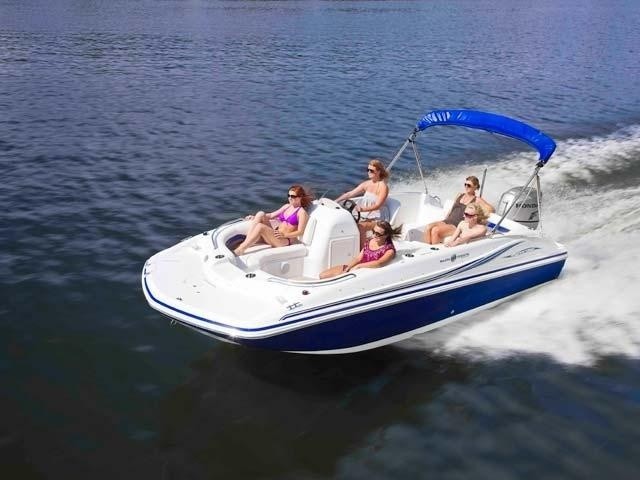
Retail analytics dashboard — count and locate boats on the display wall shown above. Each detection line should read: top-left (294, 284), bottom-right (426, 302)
top-left (142, 109), bottom-right (568, 356)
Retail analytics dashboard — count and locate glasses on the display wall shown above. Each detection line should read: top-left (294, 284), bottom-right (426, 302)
top-left (464, 212), bottom-right (475, 218)
top-left (371, 228), bottom-right (384, 237)
top-left (464, 182), bottom-right (476, 188)
top-left (367, 168), bottom-right (379, 173)
top-left (287, 194), bottom-right (300, 198)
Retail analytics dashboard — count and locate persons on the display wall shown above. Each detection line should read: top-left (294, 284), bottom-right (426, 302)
top-left (332, 159), bottom-right (392, 250)
top-left (423, 175), bottom-right (497, 245)
top-left (318, 219), bottom-right (403, 280)
top-left (442, 202), bottom-right (488, 247)
top-left (230, 184), bottom-right (316, 257)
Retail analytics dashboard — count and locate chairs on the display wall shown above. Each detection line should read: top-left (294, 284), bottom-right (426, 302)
top-left (242, 215), bottom-right (317, 277)
top-left (366, 197), bottom-right (401, 237)
